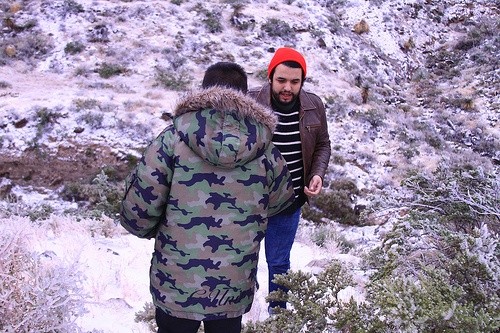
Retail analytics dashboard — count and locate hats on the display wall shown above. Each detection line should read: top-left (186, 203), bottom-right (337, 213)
top-left (267, 48), bottom-right (306, 81)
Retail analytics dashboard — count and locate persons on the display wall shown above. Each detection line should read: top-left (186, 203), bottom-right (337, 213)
top-left (248, 48), bottom-right (331, 315)
top-left (119, 62), bottom-right (296, 333)
